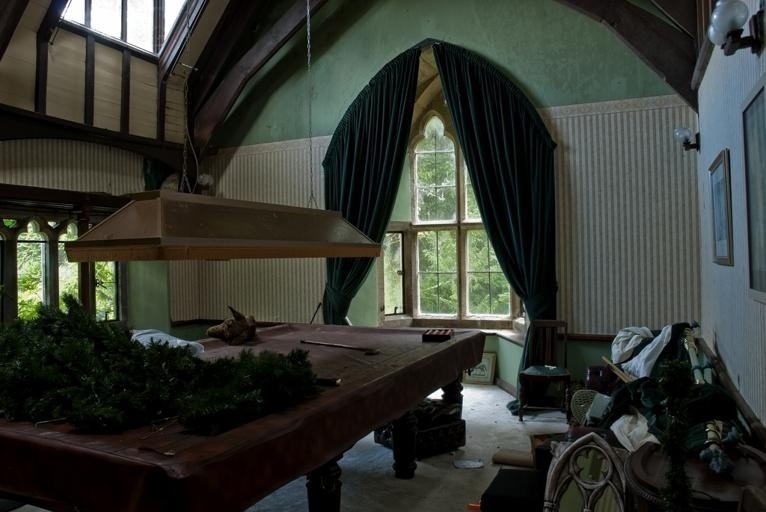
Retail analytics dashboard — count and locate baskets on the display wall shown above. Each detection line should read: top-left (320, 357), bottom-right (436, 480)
top-left (570, 389), bottom-right (599, 426)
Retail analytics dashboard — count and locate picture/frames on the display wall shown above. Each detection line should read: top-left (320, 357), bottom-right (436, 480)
top-left (463, 353), bottom-right (496, 385)
top-left (708, 147), bottom-right (734, 267)
top-left (738, 73), bottom-right (766, 304)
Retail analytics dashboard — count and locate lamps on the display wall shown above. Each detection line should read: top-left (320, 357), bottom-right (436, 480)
top-left (674, 125), bottom-right (701, 153)
top-left (705, 0), bottom-right (764, 55)
top-left (66, 1), bottom-right (380, 262)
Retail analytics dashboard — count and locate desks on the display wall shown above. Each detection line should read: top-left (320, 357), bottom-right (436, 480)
top-left (0, 320), bottom-right (487, 512)
top-left (623, 436), bottom-right (766, 512)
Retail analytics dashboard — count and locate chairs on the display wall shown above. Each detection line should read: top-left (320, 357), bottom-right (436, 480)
top-left (518, 319), bottom-right (573, 424)
